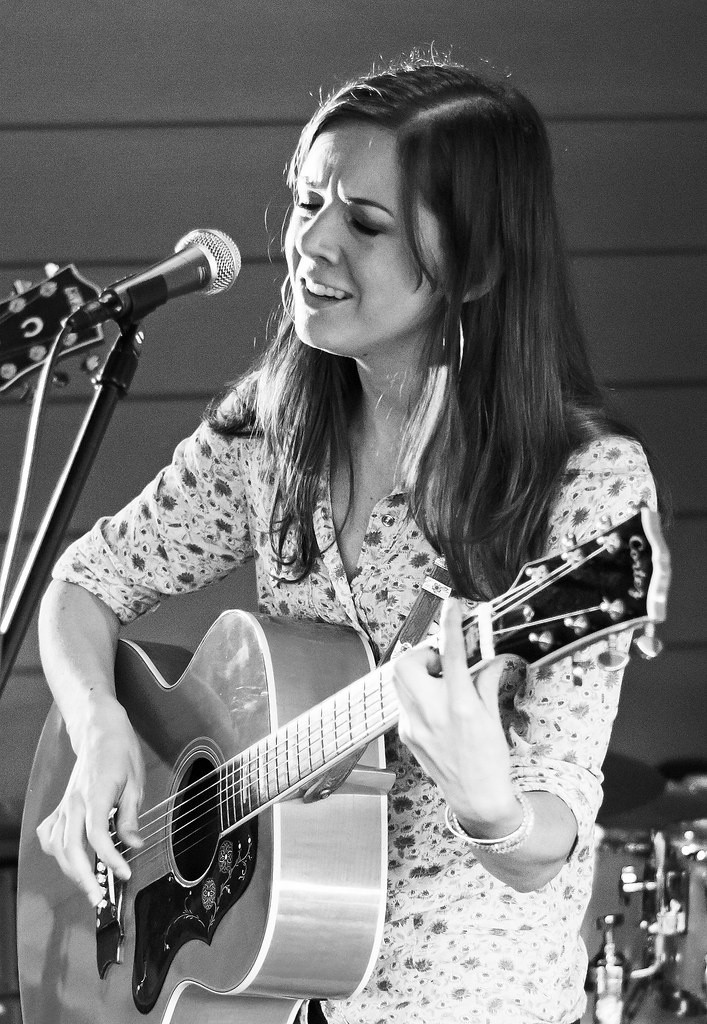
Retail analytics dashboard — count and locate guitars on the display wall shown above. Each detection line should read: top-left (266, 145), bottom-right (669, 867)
top-left (8, 499), bottom-right (681, 1024)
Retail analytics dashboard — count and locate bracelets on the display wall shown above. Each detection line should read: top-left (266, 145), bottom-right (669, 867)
top-left (446, 787), bottom-right (535, 853)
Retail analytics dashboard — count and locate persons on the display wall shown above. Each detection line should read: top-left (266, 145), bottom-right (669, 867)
top-left (37, 61), bottom-right (659, 1024)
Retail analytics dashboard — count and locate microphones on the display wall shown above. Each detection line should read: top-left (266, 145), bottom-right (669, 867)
top-left (67, 229), bottom-right (241, 333)
